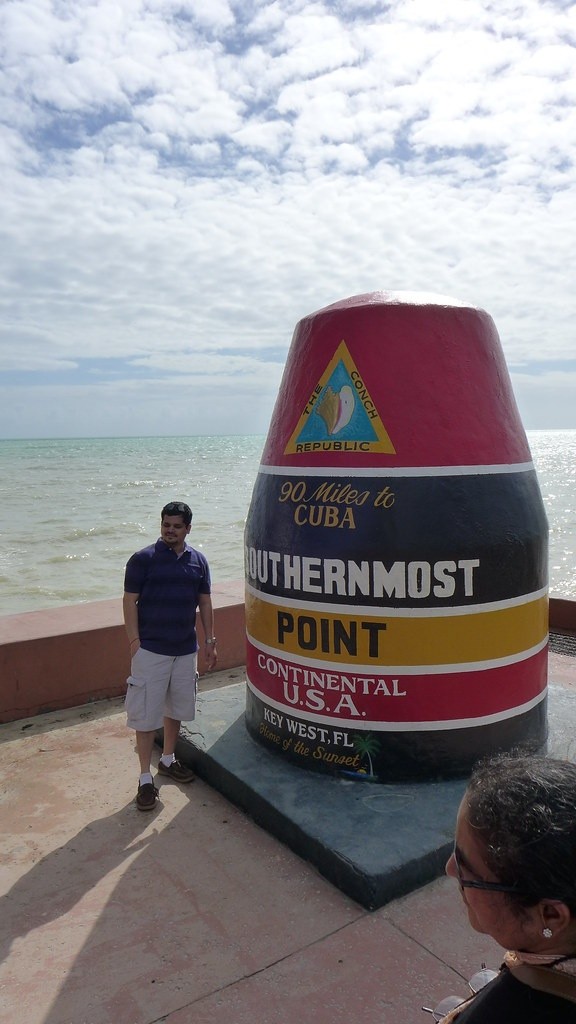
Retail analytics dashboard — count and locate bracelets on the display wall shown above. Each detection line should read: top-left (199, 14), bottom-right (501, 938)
top-left (205, 636), bottom-right (217, 644)
top-left (129, 638), bottom-right (140, 645)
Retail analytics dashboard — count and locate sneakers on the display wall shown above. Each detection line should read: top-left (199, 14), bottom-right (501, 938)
top-left (137, 779), bottom-right (157, 811)
top-left (158, 760), bottom-right (195, 783)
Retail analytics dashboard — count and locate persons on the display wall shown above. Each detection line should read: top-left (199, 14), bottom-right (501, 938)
top-left (123, 502), bottom-right (215, 810)
top-left (435, 746), bottom-right (576, 1024)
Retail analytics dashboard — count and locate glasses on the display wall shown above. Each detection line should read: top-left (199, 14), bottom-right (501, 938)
top-left (453, 839), bottom-right (567, 902)
top-left (166, 503), bottom-right (184, 512)
